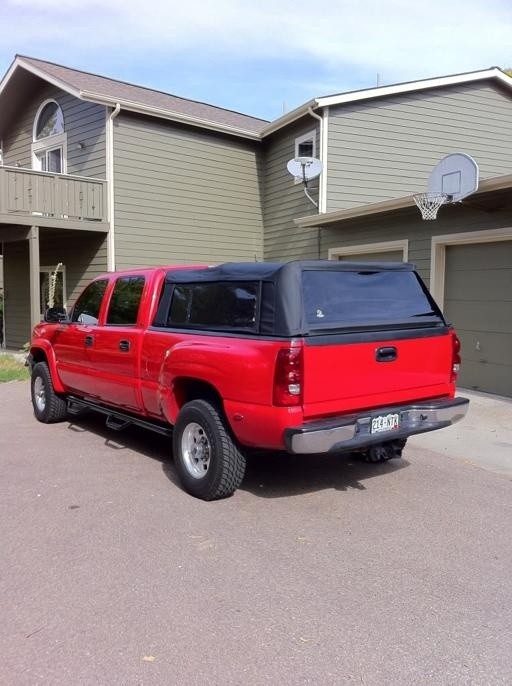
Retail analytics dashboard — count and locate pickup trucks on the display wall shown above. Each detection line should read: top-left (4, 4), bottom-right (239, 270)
top-left (25, 257), bottom-right (472, 502)
